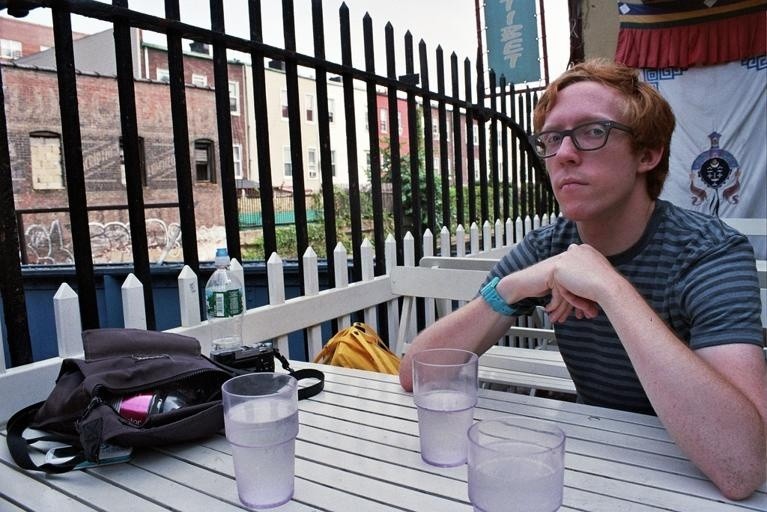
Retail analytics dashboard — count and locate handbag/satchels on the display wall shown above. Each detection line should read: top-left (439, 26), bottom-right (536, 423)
top-left (6, 327), bottom-right (228, 474)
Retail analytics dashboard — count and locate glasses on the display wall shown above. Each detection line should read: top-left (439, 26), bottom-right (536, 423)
top-left (528, 120), bottom-right (634, 159)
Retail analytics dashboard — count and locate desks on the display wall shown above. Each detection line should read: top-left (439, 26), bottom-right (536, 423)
top-left (0, 349), bottom-right (767, 512)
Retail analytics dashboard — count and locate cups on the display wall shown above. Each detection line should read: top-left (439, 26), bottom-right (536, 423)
top-left (224, 372), bottom-right (299, 508)
top-left (410, 349), bottom-right (480, 464)
top-left (468, 420), bottom-right (565, 512)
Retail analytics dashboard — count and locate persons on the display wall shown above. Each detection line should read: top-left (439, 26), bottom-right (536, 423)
top-left (400, 58), bottom-right (767, 500)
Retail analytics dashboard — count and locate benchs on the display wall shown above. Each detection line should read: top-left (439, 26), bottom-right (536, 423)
top-left (386, 247), bottom-right (580, 399)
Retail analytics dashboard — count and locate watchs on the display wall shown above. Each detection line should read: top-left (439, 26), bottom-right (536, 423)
top-left (480, 276), bottom-right (516, 316)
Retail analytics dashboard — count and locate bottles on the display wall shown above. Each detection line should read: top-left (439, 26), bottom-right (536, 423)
top-left (204, 248), bottom-right (244, 350)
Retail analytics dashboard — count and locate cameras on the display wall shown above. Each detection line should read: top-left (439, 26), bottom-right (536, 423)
top-left (210, 344), bottom-right (274, 376)
